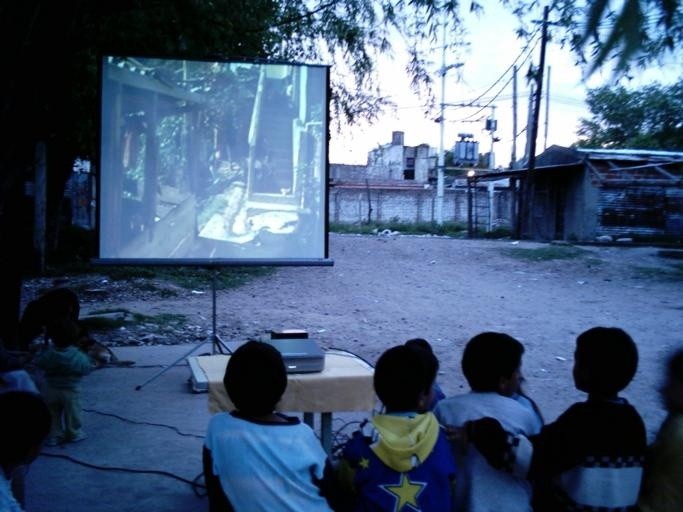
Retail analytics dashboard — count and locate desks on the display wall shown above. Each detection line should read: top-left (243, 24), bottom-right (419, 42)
top-left (187, 351), bottom-right (374, 462)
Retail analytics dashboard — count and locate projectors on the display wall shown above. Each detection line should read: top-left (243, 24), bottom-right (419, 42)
top-left (262, 338), bottom-right (326, 373)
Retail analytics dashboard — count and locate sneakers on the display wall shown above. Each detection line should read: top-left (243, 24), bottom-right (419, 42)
top-left (67, 428), bottom-right (88, 444)
top-left (44, 435), bottom-right (64, 447)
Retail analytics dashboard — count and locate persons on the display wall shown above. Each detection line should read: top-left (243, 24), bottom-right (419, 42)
top-left (433, 331), bottom-right (546, 510)
top-left (332, 337), bottom-right (472, 511)
top-left (0, 286), bottom-right (88, 510)
top-left (641, 346), bottom-right (681, 511)
top-left (201, 339), bottom-right (334, 510)
top-left (460, 325), bottom-right (649, 512)
